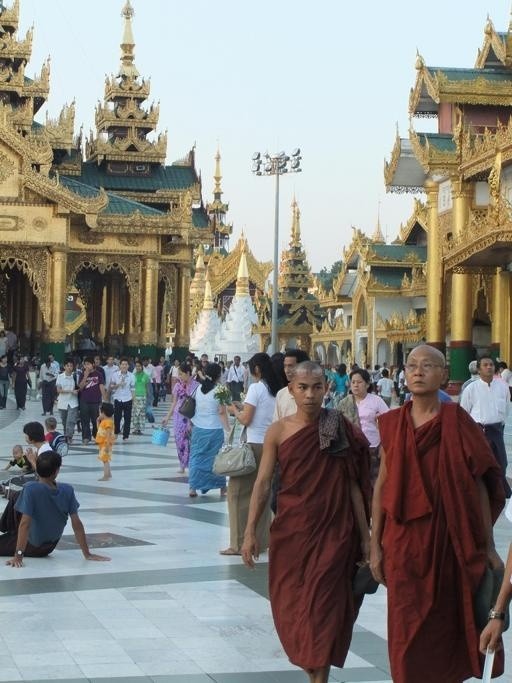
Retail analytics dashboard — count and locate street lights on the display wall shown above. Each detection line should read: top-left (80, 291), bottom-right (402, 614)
top-left (251, 144), bottom-right (306, 358)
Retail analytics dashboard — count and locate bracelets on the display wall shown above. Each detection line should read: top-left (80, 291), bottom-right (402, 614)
top-left (486, 609), bottom-right (506, 620)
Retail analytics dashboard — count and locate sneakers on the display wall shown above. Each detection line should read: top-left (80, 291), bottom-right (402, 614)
top-left (67, 427), bottom-right (144, 446)
top-left (1, 405), bottom-right (54, 417)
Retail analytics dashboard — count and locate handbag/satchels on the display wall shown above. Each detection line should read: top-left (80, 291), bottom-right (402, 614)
top-left (145, 407), bottom-right (155, 423)
top-left (212, 444), bottom-right (256, 478)
top-left (472, 564), bottom-right (508, 634)
top-left (150, 426), bottom-right (170, 448)
top-left (179, 397), bottom-right (197, 418)
top-left (158, 390), bottom-right (167, 399)
top-left (353, 562), bottom-right (381, 597)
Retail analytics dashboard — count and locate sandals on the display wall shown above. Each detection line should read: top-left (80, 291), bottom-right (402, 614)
top-left (219, 544), bottom-right (247, 556)
top-left (188, 486), bottom-right (228, 497)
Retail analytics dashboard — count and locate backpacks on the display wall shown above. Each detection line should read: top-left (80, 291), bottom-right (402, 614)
top-left (48, 433), bottom-right (69, 457)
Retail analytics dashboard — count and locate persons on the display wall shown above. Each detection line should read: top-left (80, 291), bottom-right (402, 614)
top-left (367, 346), bottom-right (512, 683)
top-left (241, 362), bottom-right (378, 683)
top-left (480, 546), bottom-right (512, 654)
top-left (2, 327), bottom-right (512, 567)
